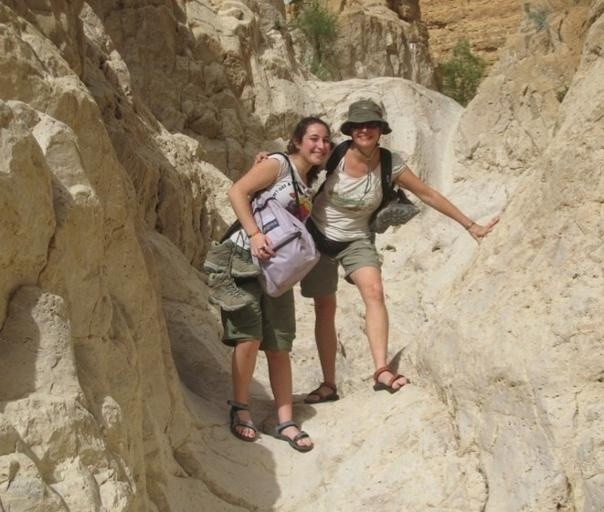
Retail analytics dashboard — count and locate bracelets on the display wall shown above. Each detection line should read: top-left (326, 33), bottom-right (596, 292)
top-left (465, 221), bottom-right (475, 229)
top-left (246, 228), bottom-right (261, 238)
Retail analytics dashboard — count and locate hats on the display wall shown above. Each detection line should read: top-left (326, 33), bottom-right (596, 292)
top-left (340, 100), bottom-right (392, 136)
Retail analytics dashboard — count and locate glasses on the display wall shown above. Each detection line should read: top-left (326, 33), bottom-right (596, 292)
top-left (349, 122), bottom-right (381, 128)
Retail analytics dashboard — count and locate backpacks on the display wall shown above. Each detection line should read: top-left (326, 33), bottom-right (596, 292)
top-left (241, 152), bottom-right (320, 298)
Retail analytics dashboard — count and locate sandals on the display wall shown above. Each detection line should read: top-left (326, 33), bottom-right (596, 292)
top-left (304, 383), bottom-right (339, 403)
top-left (373, 367), bottom-right (410, 392)
top-left (226, 399), bottom-right (257, 441)
top-left (274, 421), bottom-right (313, 451)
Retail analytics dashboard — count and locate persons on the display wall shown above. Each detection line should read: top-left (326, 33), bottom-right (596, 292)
top-left (253, 99), bottom-right (501, 405)
top-left (205, 114), bottom-right (329, 452)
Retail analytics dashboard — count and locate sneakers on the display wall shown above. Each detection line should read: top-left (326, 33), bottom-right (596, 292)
top-left (372, 189), bottom-right (420, 233)
top-left (207, 272), bottom-right (253, 312)
top-left (203, 239), bottom-right (261, 277)
